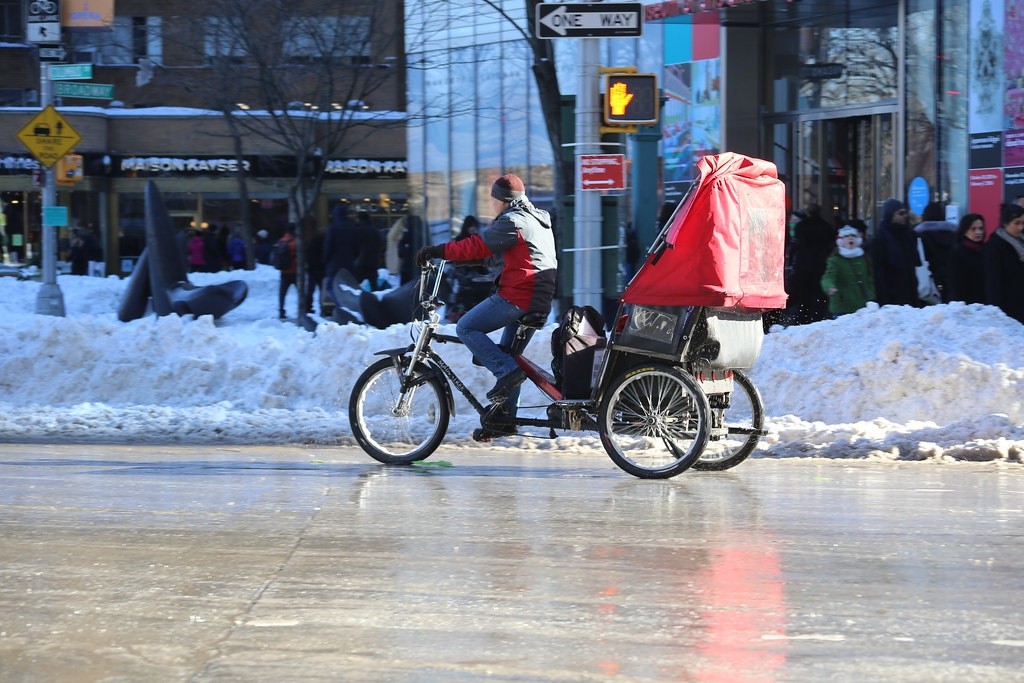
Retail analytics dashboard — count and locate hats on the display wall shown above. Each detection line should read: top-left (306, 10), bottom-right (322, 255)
top-left (491, 174), bottom-right (525, 203)
top-left (257, 229), bottom-right (269, 238)
top-left (879, 198), bottom-right (906, 223)
top-left (923, 200), bottom-right (950, 216)
top-left (792, 208), bottom-right (811, 219)
top-left (835, 223), bottom-right (859, 237)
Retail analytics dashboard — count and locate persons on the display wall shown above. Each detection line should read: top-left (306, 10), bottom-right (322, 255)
top-left (278, 202), bottom-right (387, 319)
top-left (455, 212), bottom-right (489, 276)
top-left (178, 220), bottom-right (250, 271)
top-left (67, 220), bottom-right (101, 276)
top-left (985, 202), bottom-right (1024, 324)
top-left (118, 227), bottom-right (132, 256)
top-left (943, 212), bottom-right (996, 307)
top-left (788, 201), bottom-right (874, 322)
top-left (867, 196), bottom-right (931, 309)
top-left (399, 215), bottom-right (431, 284)
top-left (624, 221), bottom-right (641, 280)
top-left (913, 199), bottom-right (961, 292)
top-left (248, 229), bottom-right (279, 269)
top-left (415, 173), bottom-right (559, 436)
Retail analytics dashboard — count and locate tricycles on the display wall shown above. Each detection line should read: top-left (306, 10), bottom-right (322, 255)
top-left (349, 151), bottom-right (789, 480)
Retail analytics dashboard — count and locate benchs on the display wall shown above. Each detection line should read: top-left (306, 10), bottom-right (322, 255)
top-left (608, 152), bottom-right (790, 371)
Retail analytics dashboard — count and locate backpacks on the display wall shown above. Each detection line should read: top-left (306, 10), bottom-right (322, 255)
top-left (270, 237), bottom-right (297, 269)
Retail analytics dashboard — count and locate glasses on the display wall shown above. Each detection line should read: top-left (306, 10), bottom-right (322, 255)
top-left (894, 211), bottom-right (909, 216)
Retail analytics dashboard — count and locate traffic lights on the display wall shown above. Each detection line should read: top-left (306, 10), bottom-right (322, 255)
top-left (605, 73), bottom-right (657, 124)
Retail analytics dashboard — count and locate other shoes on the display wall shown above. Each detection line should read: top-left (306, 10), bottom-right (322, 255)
top-left (279, 309), bottom-right (287, 318)
top-left (308, 310), bottom-right (315, 313)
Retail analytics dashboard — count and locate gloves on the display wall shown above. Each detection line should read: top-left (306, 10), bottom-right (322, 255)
top-left (416, 243), bottom-right (445, 268)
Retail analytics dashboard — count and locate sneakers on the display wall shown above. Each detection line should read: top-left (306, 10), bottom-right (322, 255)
top-left (473, 425), bottom-right (518, 438)
top-left (486, 367), bottom-right (527, 399)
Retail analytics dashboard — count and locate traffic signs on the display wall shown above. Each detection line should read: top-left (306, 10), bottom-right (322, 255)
top-left (536, 2), bottom-right (644, 40)
